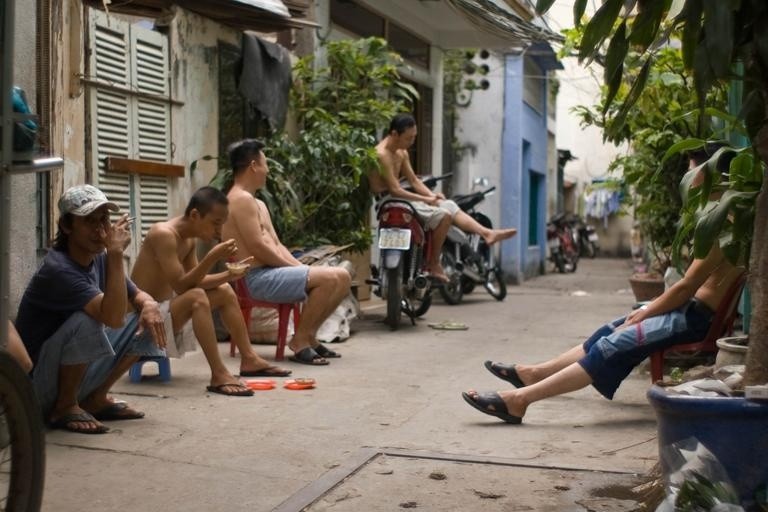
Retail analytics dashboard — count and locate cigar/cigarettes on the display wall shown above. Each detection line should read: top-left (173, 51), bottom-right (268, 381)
top-left (126, 216), bottom-right (137, 222)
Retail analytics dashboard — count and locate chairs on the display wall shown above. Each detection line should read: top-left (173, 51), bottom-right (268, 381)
top-left (226, 254), bottom-right (301, 360)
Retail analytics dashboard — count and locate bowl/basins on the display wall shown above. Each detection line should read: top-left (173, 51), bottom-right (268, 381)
top-left (227, 263), bottom-right (248, 275)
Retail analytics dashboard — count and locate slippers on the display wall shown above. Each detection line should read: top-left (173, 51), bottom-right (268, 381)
top-left (428, 319), bottom-right (469, 330)
top-left (289, 343), bottom-right (341, 365)
top-left (239, 364), bottom-right (288, 376)
top-left (206, 382), bottom-right (254, 396)
top-left (91, 398), bottom-right (145, 420)
top-left (484, 359), bottom-right (525, 388)
top-left (461, 390), bottom-right (522, 424)
top-left (48, 411), bottom-right (106, 434)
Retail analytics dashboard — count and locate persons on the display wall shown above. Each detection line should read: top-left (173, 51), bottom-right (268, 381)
top-left (370, 113), bottom-right (517, 284)
top-left (5, 317), bottom-right (34, 373)
top-left (15, 184), bottom-right (169, 434)
top-left (221, 140), bottom-right (352, 366)
top-left (127, 187), bottom-right (292, 397)
top-left (463, 141), bottom-right (746, 423)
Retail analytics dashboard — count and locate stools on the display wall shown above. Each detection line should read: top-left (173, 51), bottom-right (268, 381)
top-left (130, 356), bottom-right (170, 383)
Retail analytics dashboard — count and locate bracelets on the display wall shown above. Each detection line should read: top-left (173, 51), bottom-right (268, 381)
top-left (640, 305), bottom-right (647, 311)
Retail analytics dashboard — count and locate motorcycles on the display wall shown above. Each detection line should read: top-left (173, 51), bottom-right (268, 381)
top-left (547, 212), bottom-right (599, 272)
top-left (437, 186), bottom-right (506, 303)
top-left (365, 173), bottom-right (453, 325)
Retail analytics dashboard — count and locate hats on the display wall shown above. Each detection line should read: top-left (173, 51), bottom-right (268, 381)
top-left (58, 185), bottom-right (119, 218)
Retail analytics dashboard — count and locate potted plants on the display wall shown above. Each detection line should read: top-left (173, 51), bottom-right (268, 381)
top-left (531, 0), bottom-right (768, 512)
